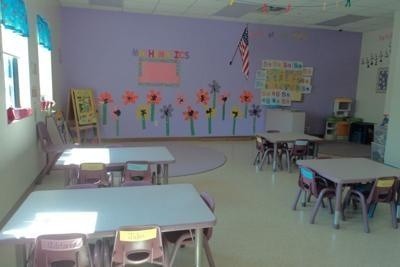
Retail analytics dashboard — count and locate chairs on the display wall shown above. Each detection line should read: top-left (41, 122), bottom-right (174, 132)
top-left (291, 163), bottom-right (336, 224)
top-left (121, 160), bottom-right (156, 186)
top-left (342, 176), bottom-right (398, 233)
top-left (36, 121), bottom-right (80, 184)
top-left (102, 225), bottom-right (168, 267)
top-left (164, 192), bottom-right (215, 267)
top-left (79, 163), bottom-right (112, 188)
top-left (30, 233), bottom-right (101, 267)
top-left (253, 134), bottom-right (283, 170)
top-left (286, 138), bottom-right (309, 171)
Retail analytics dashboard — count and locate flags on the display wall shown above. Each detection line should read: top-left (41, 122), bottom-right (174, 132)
top-left (238, 25), bottom-right (249, 79)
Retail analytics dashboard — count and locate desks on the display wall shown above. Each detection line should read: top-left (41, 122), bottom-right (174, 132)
top-left (295, 157), bottom-right (400, 228)
top-left (255, 133), bottom-right (324, 172)
top-left (53, 145), bottom-right (175, 185)
top-left (0, 183), bottom-right (216, 266)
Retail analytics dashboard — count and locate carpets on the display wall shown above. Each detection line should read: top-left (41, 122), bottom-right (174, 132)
top-left (47, 140), bottom-right (227, 177)
